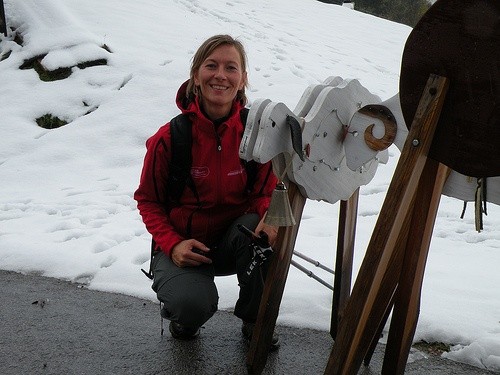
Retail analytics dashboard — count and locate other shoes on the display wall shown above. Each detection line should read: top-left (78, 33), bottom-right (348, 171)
top-left (169, 321), bottom-right (191, 339)
top-left (242, 320), bottom-right (279, 348)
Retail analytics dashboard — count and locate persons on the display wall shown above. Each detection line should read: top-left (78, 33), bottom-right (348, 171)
top-left (133, 34), bottom-right (294, 345)
